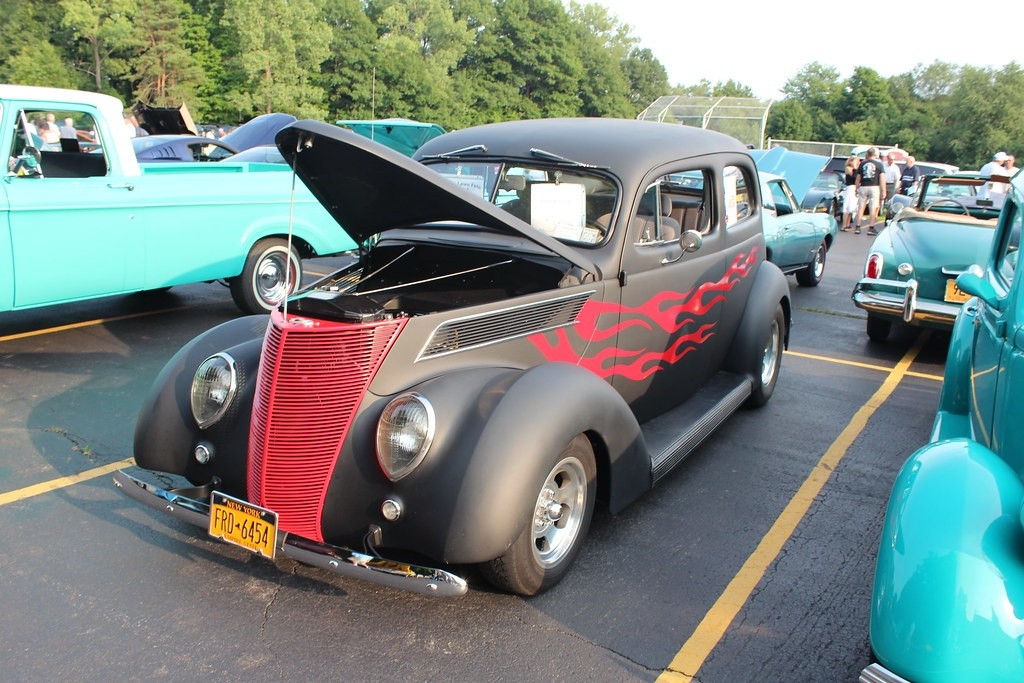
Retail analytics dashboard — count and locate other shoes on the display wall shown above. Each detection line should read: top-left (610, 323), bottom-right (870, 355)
top-left (841, 226), bottom-right (853, 231)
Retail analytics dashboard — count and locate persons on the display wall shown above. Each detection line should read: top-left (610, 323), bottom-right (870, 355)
top-left (841, 147), bottom-right (919, 235)
top-left (25, 114), bottom-right (148, 153)
top-left (979, 152), bottom-right (1020, 191)
top-left (206, 128), bottom-right (227, 146)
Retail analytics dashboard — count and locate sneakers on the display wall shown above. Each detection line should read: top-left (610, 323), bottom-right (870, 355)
top-left (867, 228), bottom-right (878, 236)
top-left (855, 228), bottom-right (861, 234)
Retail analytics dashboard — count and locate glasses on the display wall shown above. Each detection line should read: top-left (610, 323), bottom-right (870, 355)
top-left (888, 158), bottom-right (895, 160)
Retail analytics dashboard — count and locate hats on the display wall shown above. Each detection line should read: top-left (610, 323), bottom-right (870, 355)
top-left (993, 152), bottom-right (1009, 161)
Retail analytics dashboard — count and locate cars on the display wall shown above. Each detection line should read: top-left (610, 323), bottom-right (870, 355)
top-left (851, 172), bottom-right (1011, 341)
top-left (655, 146), bottom-right (840, 287)
top-left (800, 145), bottom-right (979, 227)
top-left (0, 82), bottom-right (363, 314)
top-left (858, 167), bottom-right (1024, 683)
top-left (112, 116), bottom-right (793, 599)
